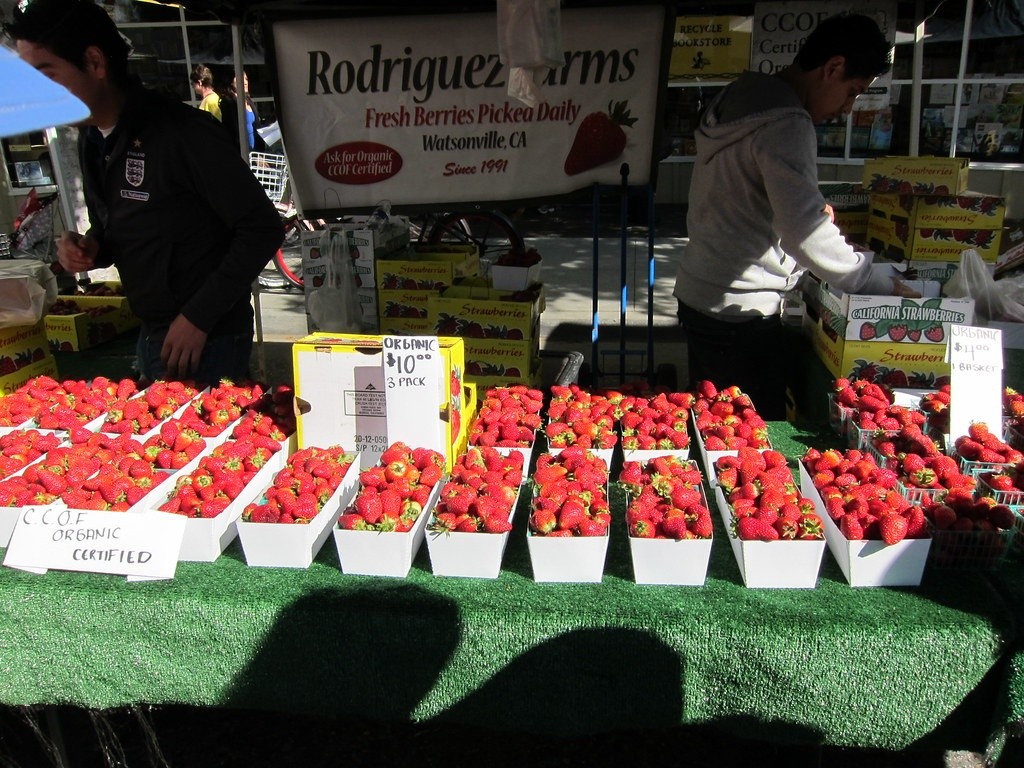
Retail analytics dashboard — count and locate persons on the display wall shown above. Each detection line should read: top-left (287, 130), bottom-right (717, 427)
top-left (189, 63), bottom-right (222, 122)
top-left (672, 14), bottom-right (923, 420)
top-left (219, 69), bottom-right (271, 169)
top-left (10, 1), bottom-right (286, 386)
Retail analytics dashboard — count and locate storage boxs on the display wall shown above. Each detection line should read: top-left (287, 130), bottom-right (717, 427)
top-left (3, 160), bottom-right (1022, 592)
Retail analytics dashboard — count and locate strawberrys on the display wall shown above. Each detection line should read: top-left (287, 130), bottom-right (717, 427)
top-left (0, 375), bottom-right (1023, 577)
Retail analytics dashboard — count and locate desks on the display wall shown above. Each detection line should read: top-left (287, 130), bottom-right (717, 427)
top-left (2, 410), bottom-right (1024, 768)
